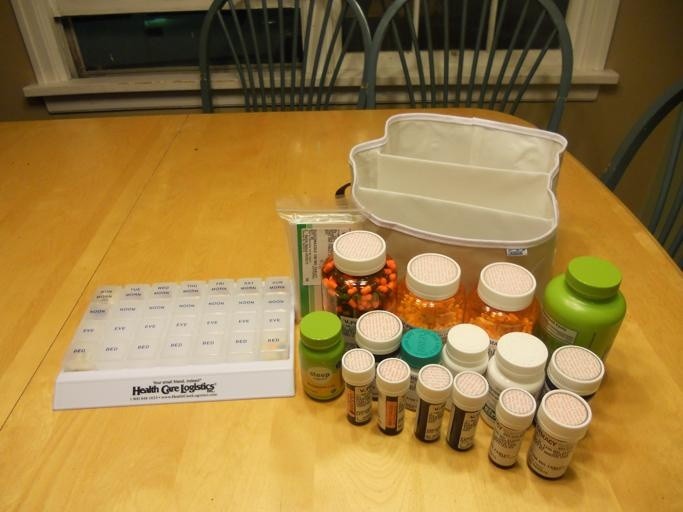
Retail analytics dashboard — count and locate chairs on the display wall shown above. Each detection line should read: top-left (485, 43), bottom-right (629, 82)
top-left (366, 0), bottom-right (575, 134)
top-left (198, 0), bottom-right (373, 107)
top-left (600, 81), bottom-right (683, 271)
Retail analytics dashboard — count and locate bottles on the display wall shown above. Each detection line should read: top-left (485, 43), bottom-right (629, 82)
top-left (297, 229), bottom-right (628, 480)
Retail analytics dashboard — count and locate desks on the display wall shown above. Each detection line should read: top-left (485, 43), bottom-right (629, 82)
top-left (0, 106), bottom-right (682, 511)
